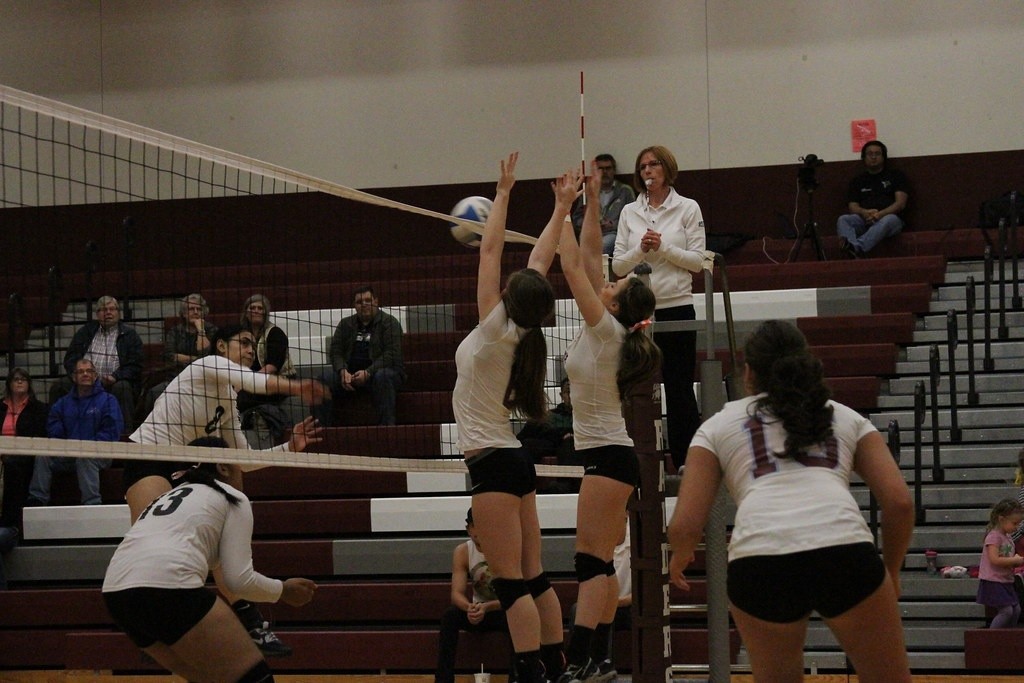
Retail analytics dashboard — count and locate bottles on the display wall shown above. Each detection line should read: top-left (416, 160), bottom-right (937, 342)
top-left (926, 552), bottom-right (937, 573)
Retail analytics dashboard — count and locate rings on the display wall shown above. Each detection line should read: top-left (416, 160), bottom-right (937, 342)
top-left (648, 238), bottom-right (653, 244)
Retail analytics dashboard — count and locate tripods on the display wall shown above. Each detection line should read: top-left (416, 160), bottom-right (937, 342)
top-left (790, 181), bottom-right (827, 264)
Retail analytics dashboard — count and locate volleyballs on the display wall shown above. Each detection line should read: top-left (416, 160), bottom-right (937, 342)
top-left (449, 196), bottom-right (494, 250)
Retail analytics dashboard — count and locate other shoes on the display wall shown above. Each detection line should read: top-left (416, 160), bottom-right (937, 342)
top-left (671, 447), bottom-right (686, 474)
top-left (567, 657), bottom-right (600, 683)
top-left (840, 247), bottom-right (862, 259)
top-left (595, 659), bottom-right (617, 683)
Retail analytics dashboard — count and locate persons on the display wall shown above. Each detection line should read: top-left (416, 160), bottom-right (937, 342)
top-left (309, 286), bottom-right (408, 429)
top-left (837, 141), bottom-right (912, 261)
top-left (103, 434), bottom-right (317, 683)
top-left (666, 320), bottom-right (916, 683)
top-left (451, 151), bottom-right (579, 683)
top-left (544, 378), bottom-right (585, 464)
top-left (974, 447), bottom-right (1024, 631)
top-left (571, 153), bottom-right (634, 257)
top-left (613, 143), bottom-right (705, 475)
top-left (435, 506), bottom-right (521, 683)
top-left (0, 293), bottom-right (299, 524)
top-left (549, 159), bottom-right (661, 683)
top-left (122, 322), bottom-right (322, 657)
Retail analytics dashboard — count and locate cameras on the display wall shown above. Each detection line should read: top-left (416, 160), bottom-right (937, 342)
top-left (795, 154), bottom-right (825, 185)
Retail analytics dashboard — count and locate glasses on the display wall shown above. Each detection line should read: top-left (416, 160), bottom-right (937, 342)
top-left (640, 160), bottom-right (663, 170)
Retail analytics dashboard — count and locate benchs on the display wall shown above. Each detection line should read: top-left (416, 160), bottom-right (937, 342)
top-left (0, 225), bottom-right (1024, 672)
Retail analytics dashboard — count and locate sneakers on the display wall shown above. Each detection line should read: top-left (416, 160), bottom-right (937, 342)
top-left (247, 620), bottom-right (291, 656)
